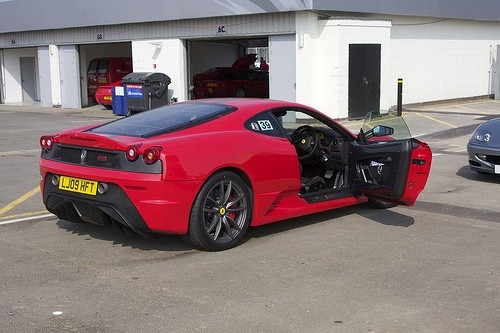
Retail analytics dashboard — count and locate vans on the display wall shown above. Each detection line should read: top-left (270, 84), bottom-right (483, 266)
top-left (86, 56), bottom-right (133, 104)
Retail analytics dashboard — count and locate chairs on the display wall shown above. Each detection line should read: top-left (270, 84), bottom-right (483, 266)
top-left (299, 155), bottom-right (324, 194)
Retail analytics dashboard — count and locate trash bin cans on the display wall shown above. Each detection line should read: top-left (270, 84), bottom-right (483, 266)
top-left (111, 71), bottom-right (171, 116)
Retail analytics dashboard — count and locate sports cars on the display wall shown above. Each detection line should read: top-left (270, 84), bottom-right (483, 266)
top-left (37, 96), bottom-right (433, 253)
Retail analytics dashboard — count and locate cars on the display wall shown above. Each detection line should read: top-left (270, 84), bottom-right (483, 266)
top-left (193, 66), bottom-right (232, 85)
top-left (192, 53), bottom-right (269, 98)
top-left (95, 79), bottom-right (123, 110)
